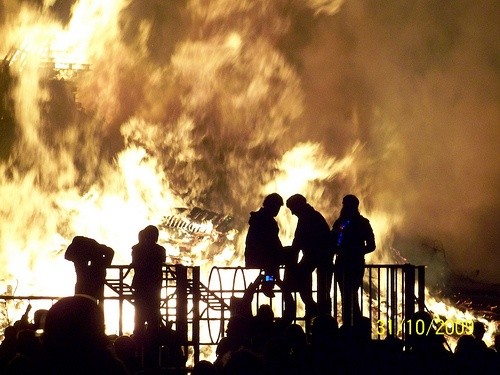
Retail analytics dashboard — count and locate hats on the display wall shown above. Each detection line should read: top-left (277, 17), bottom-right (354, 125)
top-left (342, 194), bottom-right (360, 205)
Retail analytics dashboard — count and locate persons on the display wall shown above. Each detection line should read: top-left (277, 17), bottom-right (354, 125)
top-left (65, 236), bottom-right (115, 301)
top-left (192, 273), bottom-right (500, 375)
top-left (0, 293), bottom-right (188, 375)
top-left (331, 194), bottom-right (376, 326)
top-left (131, 225), bottom-right (166, 335)
top-left (287, 194), bottom-right (332, 321)
top-left (245, 194), bottom-right (293, 302)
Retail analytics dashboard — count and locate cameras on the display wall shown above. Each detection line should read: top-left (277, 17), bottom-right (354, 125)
top-left (262, 274), bottom-right (275, 284)
